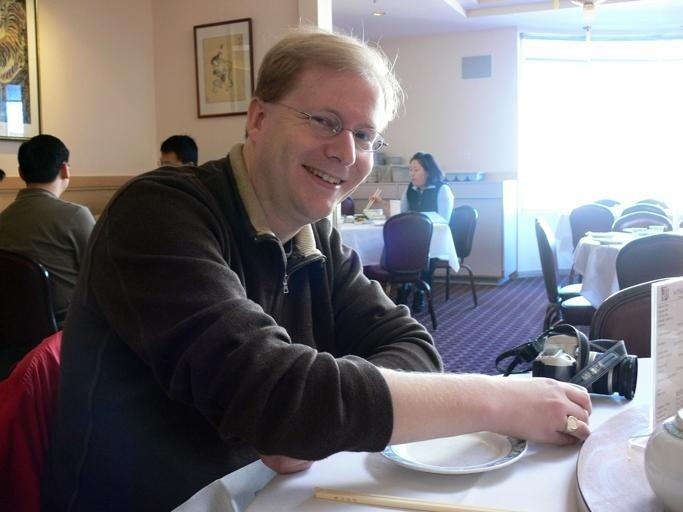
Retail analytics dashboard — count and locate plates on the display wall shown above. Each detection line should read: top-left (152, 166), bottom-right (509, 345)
top-left (378, 426), bottom-right (530, 476)
top-left (587, 229), bottom-right (656, 247)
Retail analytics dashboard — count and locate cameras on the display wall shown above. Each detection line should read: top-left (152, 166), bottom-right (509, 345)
top-left (532, 334), bottom-right (638, 400)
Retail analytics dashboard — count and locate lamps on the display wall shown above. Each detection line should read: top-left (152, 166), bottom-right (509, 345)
top-left (569, 0), bottom-right (607, 29)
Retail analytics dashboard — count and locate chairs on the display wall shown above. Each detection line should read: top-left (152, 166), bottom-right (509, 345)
top-left (0, 249), bottom-right (58, 381)
top-left (325, 193), bottom-right (478, 331)
top-left (0, 331), bottom-right (63, 512)
top-left (531, 200), bottom-right (683, 359)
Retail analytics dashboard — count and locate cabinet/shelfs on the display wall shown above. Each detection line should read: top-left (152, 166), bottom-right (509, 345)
top-left (352, 181), bottom-right (516, 286)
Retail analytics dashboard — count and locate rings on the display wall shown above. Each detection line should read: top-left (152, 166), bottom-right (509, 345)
top-left (564, 416), bottom-right (579, 434)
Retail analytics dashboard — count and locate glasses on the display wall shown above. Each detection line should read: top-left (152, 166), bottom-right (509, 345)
top-left (269, 103), bottom-right (389, 151)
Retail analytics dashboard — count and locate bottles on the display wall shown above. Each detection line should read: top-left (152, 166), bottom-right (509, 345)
top-left (641, 410), bottom-right (682, 510)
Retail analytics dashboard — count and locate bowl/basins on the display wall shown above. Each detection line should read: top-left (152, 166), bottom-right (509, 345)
top-left (347, 208), bottom-right (386, 224)
top-left (445, 172), bottom-right (481, 183)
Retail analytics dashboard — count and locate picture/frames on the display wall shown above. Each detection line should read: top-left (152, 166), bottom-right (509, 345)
top-left (0, 0), bottom-right (43, 142)
top-left (192, 18), bottom-right (255, 119)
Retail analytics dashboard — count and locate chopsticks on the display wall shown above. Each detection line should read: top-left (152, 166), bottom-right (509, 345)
top-left (311, 487), bottom-right (511, 512)
top-left (362, 187), bottom-right (382, 209)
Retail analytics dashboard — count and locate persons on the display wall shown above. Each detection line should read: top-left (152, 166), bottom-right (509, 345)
top-left (36, 27), bottom-right (591, 511)
top-left (156, 135), bottom-right (197, 168)
top-left (367, 152), bottom-right (455, 316)
top-left (0, 134), bottom-right (97, 329)
